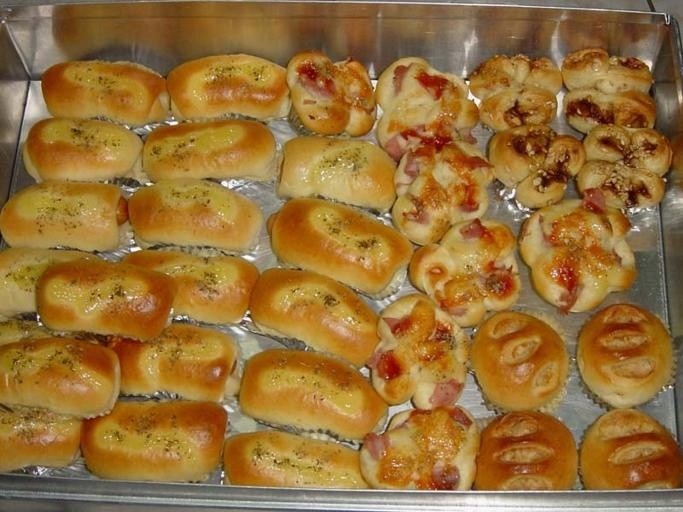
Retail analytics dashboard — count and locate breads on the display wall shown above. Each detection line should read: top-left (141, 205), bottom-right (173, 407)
top-left (1, 49), bottom-right (683, 494)
